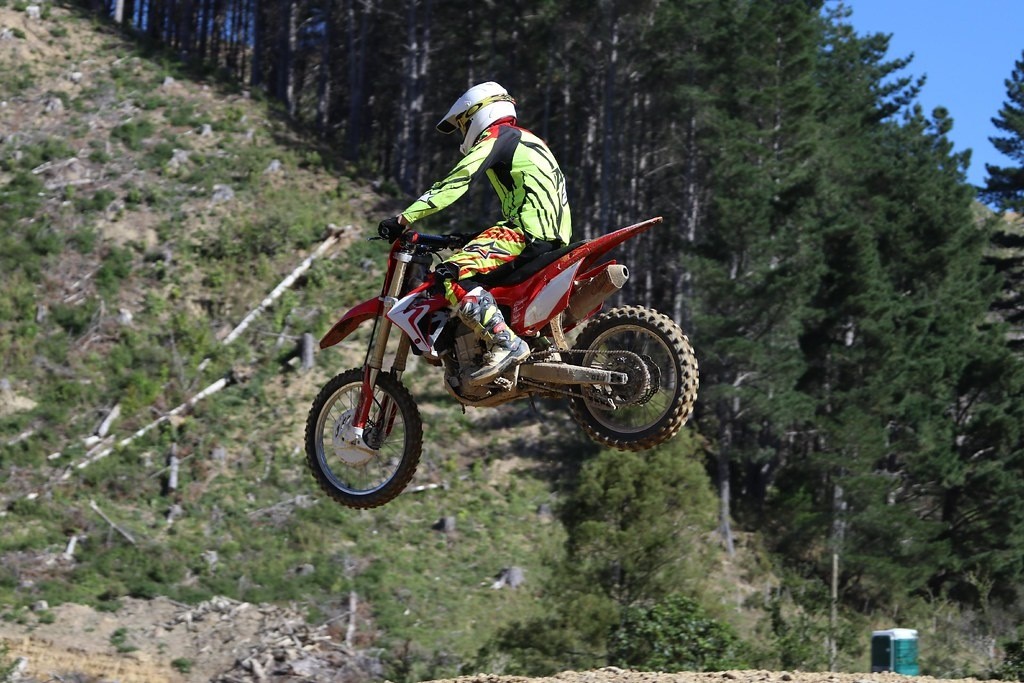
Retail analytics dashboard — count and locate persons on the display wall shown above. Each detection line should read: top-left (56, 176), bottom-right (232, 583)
top-left (379, 81), bottom-right (573, 386)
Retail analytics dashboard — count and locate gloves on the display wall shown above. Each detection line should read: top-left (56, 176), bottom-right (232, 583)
top-left (377, 215), bottom-right (406, 244)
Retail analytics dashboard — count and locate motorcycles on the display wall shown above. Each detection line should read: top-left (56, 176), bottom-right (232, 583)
top-left (303, 215), bottom-right (701, 511)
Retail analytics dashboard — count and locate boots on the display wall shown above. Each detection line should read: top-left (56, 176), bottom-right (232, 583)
top-left (453, 286), bottom-right (531, 385)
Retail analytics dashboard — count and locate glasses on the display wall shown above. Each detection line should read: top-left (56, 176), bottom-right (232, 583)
top-left (456, 93), bottom-right (516, 138)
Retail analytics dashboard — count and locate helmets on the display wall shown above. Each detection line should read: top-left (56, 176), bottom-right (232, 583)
top-left (435, 81), bottom-right (518, 156)
